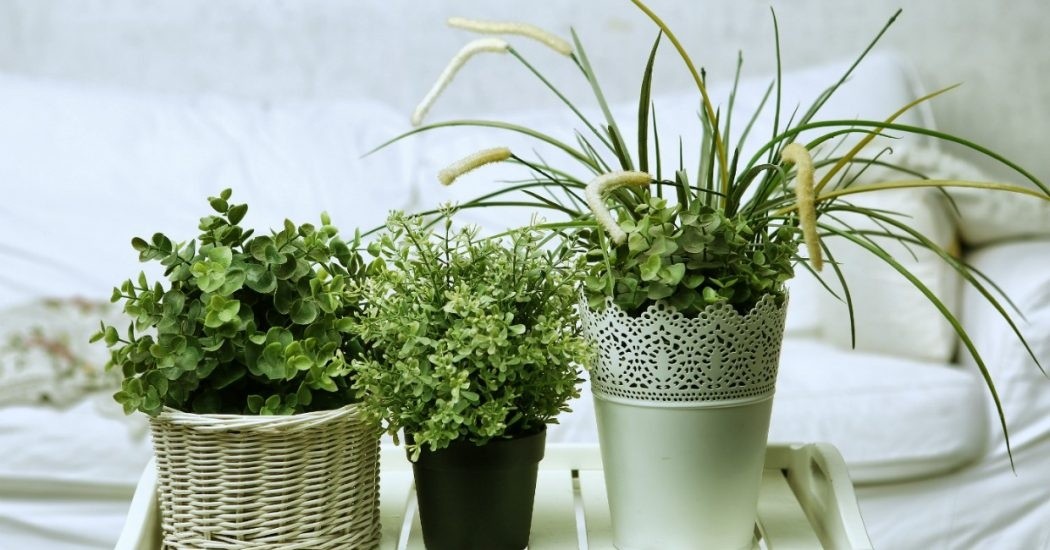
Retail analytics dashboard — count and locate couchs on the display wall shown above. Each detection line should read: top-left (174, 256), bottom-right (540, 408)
top-left (414, 52), bottom-right (1050, 550)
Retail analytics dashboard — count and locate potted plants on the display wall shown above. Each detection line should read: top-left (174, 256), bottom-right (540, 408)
top-left (90, 1), bottom-right (1050, 548)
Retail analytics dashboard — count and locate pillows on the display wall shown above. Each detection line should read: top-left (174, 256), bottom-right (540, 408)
top-left (769, 131), bottom-right (1050, 478)
